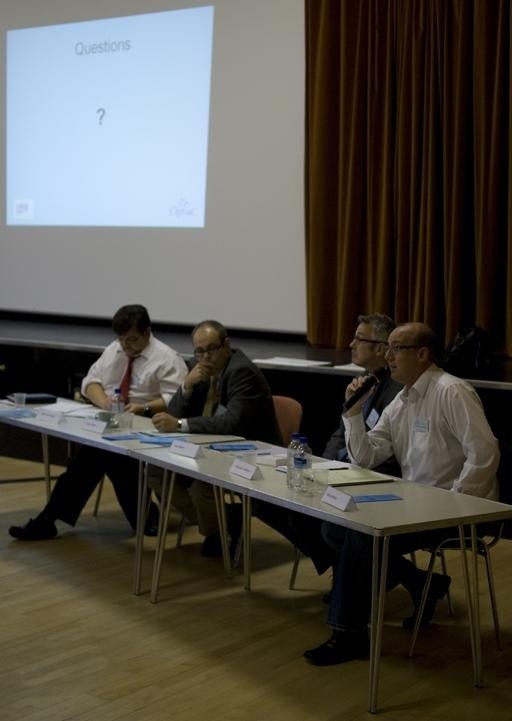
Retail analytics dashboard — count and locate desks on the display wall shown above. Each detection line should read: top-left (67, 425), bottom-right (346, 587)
top-left (130, 438), bottom-right (512, 712)
top-left (0, 396), bottom-right (236, 596)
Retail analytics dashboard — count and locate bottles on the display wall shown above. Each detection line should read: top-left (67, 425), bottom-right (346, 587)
top-left (110, 388), bottom-right (126, 432)
top-left (295, 434), bottom-right (313, 497)
top-left (287, 432), bottom-right (302, 489)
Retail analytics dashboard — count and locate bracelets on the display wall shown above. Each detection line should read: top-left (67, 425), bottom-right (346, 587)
top-left (177, 418), bottom-right (182, 432)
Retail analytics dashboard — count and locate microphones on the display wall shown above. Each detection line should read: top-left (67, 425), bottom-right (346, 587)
top-left (343, 366), bottom-right (388, 411)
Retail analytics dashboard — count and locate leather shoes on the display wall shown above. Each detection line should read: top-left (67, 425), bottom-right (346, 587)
top-left (224, 501), bottom-right (245, 564)
top-left (302, 629), bottom-right (370, 668)
top-left (8, 517), bottom-right (59, 540)
top-left (144, 502), bottom-right (162, 537)
top-left (198, 531), bottom-right (222, 560)
top-left (402, 569), bottom-right (452, 633)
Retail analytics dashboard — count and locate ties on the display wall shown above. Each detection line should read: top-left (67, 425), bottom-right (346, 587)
top-left (118, 355), bottom-right (141, 407)
top-left (201, 374), bottom-right (219, 419)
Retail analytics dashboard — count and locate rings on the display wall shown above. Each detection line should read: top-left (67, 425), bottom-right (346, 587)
top-left (155, 423), bottom-right (160, 428)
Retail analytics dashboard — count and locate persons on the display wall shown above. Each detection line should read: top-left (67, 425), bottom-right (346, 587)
top-left (302, 320), bottom-right (501, 665)
top-left (152, 319), bottom-right (285, 556)
top-left (250, 312), bottom-right (405, 605)
top-left (8, 304), bottom-right (188, 540)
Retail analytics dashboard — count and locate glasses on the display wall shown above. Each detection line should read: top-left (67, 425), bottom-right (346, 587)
top-left (383, 344), bottom-right (419, 353)
top-left (354, 335), bottom-right (389, 347)
top-left (193, 343), bottom-right (224, 358)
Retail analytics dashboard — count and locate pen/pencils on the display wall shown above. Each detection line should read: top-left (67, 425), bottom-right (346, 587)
top-left (328, 468), bottom-right (349, 470)
top-left (258, 452), bottom-right (271, 455)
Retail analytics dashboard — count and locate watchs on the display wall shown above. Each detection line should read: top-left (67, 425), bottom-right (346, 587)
top-left (144, 403), bottom-right (152, 416)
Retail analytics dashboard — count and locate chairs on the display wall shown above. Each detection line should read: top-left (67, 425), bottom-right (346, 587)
top-left (376, 437), bottom-right (511, 655)
top-left (178, 395), bottom-right (303, 570)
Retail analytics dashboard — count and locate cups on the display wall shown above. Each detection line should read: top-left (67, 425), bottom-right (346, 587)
top-left (314, 467), bottom-right (330, 496)
top-left (120, 411), bottom-right (134, 436)
top-left (12, 391), bottom-right (27, 410)
